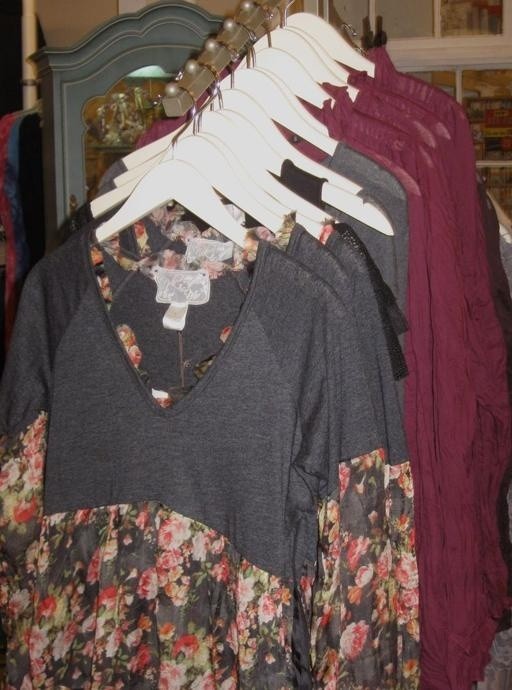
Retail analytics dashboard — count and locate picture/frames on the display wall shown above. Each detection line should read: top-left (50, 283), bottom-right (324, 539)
top-left (433, 0), bottom-right (512, 38)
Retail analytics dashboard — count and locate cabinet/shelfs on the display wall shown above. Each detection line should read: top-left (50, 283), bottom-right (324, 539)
top-left (25, 0), bottom-right (240, 268)
